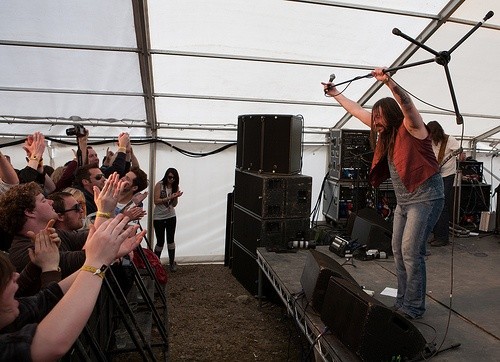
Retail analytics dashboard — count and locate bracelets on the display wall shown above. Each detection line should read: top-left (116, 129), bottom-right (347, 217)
top-left (82, 265), bottom-right (105, 279)
top-left (30, 156), bottom-right (39, 161)
top-left (119, 147), bottom-right (126, 150)
top-left (96, 212), bottom-right (110, 218)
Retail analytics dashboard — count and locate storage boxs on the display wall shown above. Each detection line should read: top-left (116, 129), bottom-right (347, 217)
top-left (231, 169), bottom-right (312, 301)
top-left (321, 128), bottom-right (378, 225)
top-left (479, 212), bottom-right (496, 232)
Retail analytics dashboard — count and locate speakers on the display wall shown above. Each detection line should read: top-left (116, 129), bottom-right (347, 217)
top-left (230, 241), bottom-right (271, 299)
top-left (279, 216), bottom-right (310, 252)
top-left (301, 250), bottom-right (361, 312)
top-left (266, 116), bottom-right (302, 175)
top-left (327, 128), bottom-right (378, 182)
top-left (231, 207), bottom-right (283, 257)
top-left (353, 218), bottom-right (393, 255)
top-left (461, 183), bottom-right (491, 224)
top-left (278, 173), bottom-right (312, 218)
top-left (321, 276), bottom-right (427, 361)
top-left (236, 114), bottom-right (268, 173)
top-left (233, 170), bottom-right (283, 218)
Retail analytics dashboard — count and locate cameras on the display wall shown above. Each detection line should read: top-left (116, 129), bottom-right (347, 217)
top-left (66, 124), bottom-right (86, 138)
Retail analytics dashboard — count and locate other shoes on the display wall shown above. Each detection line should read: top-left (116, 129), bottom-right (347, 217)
top-left (396, 310), bottom-right (410, 318)
top-left (170, 265), bottom-right (177, 272)
top-left (389, 306), bottom-right (399, 311)
top-left (428, 239), bottom-right (449, 247)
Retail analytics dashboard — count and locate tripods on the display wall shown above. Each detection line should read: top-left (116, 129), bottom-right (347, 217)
top-left (328, 10), bottom-right (495, 125)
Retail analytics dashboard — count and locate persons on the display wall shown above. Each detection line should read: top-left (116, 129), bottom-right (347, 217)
top-left (153, 168), bottom-right (183, 271)
top-left (322, 68), bottom-right (444, 316)
top-left (426, 120), bottom-right (466, 247)
top-left (0, 133), bottom-right (148, 362)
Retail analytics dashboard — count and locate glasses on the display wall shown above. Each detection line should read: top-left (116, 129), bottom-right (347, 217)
top-left (168, 176), bottom-right (175, 179)
top-left (63, 203), bottom-right (82, 212)
top-left (95, 173), bottom-right (105, 180)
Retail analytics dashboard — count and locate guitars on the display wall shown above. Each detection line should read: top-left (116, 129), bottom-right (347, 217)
top-left (438, 146), bottom-right (464, 173)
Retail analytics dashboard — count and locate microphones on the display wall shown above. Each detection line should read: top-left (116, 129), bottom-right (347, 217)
top-left (325, 74), bottom-right (336, 93)
